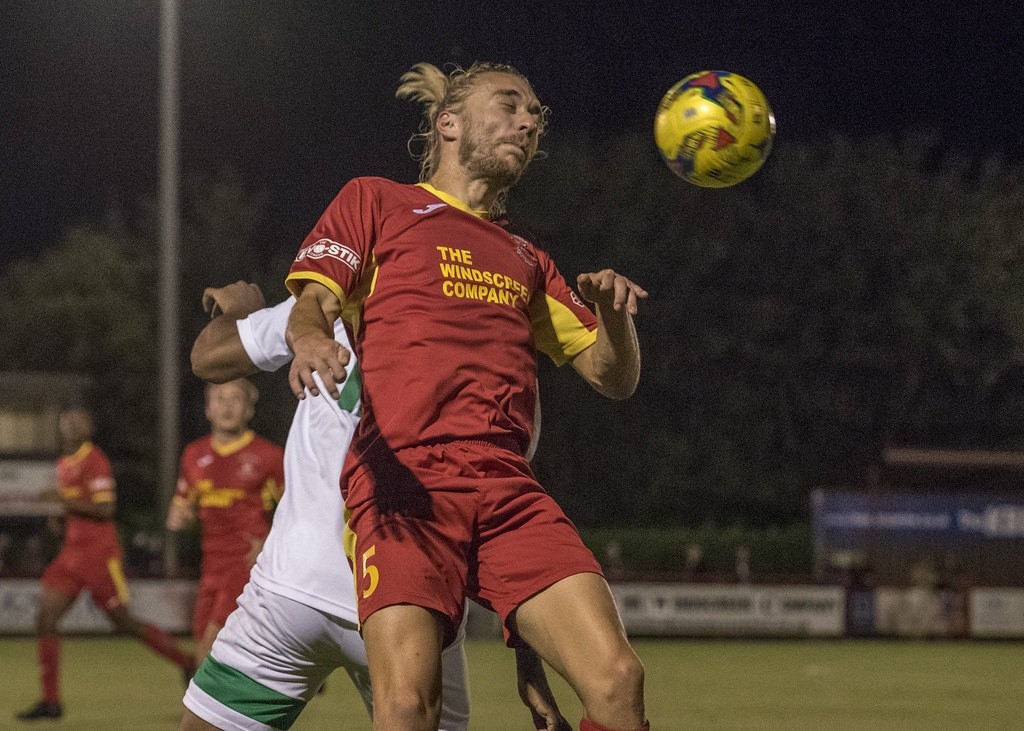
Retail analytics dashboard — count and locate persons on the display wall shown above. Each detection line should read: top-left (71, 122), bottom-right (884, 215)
top-left (15, 398), bottom-right (200, 720)
top-left (283, 57), bottom-right (650, 731)
top-left (165, 376), bottom-right (328, 694)
top-left (179, 281), bottom-right (574, 731)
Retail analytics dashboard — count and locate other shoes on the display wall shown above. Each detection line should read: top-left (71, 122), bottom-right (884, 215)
top-left (181, 660), bottom-right (197, 684)
top-left (14, 701), bottom-right (62, 721)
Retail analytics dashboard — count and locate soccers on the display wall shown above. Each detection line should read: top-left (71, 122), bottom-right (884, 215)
top-left (651, 68), bottom-right (779, 190)
